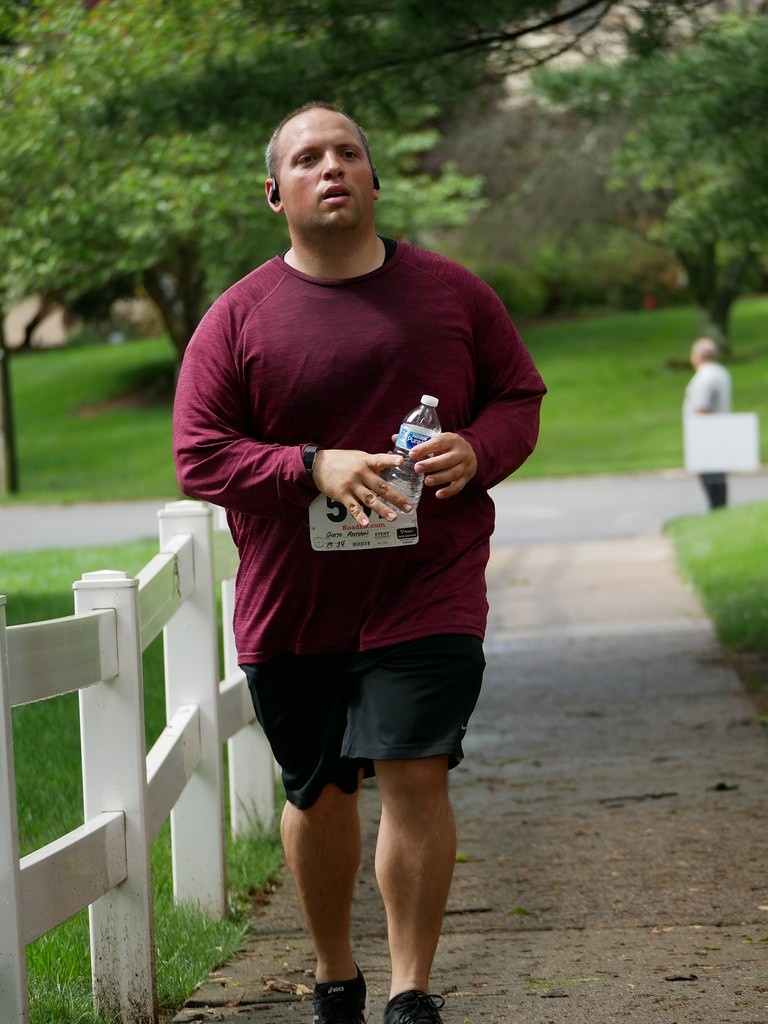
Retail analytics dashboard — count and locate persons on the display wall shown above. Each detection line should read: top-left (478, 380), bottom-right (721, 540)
top-left (682, 338), bottom-right (731, 510)
top-left (174, 102), bottom-right (546, 1024)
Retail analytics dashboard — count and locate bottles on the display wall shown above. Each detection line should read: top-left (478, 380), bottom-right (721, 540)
top-left (381, 394), bottom-right (440, 515)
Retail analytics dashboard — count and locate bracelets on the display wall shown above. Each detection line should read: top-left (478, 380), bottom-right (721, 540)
top-left (303, 444), bottom-right (319, 479)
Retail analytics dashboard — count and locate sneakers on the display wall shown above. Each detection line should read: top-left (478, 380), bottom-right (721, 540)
top-left (383, 990), bottom-right (445, 1024)
top-left (312, 959), bottom-right (367, 1024)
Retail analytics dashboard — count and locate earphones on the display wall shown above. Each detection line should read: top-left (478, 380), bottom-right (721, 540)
top-left (373, 170), bottom-right (380, 190)
top-left (270, 176), bottom-right (281, 203)
